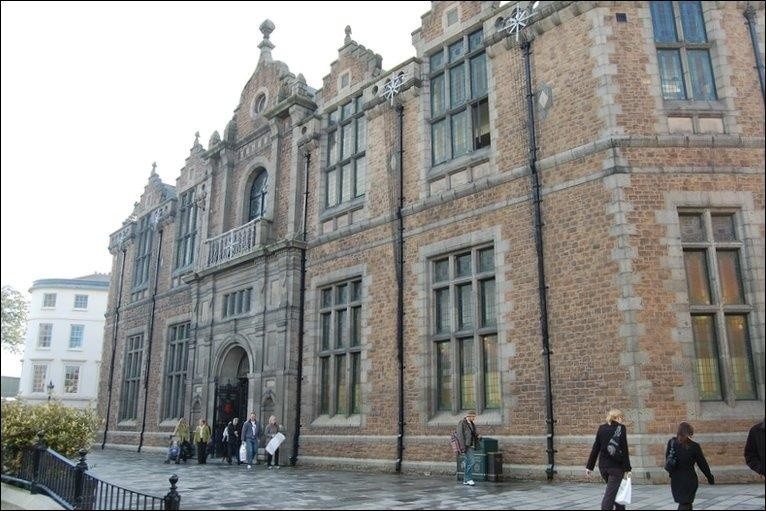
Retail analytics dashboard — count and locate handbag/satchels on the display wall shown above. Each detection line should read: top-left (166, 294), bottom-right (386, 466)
top-left (474, 437), bottom-right (481, 450)
top-left (451, 431), bottom-right (461, 455)
top-left (221, 441), bottom-right (228, 457)
top-left (665, 449), bottom-right (677, 472)
top-left (240, 445), bottom-right (246, 461)
top-left (181, 441), bottom-right (192, 458)
top-left (608, 433), bottom-right (622, 461)
top-left (614, 478), bottom-right (631, 506)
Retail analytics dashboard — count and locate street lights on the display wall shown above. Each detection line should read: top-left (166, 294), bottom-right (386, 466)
top-left (46, 380), bottom-right (55, 408)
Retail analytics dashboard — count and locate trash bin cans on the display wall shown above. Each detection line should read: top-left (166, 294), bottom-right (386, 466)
top-left (456, 437), bottom-right (503, 482)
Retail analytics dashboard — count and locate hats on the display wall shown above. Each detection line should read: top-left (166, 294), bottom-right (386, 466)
top-left (467, 411), bottom-right (477, 416)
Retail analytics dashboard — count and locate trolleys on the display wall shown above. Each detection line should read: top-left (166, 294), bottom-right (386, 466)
top-left (163, 434), bottom-right (189, 465)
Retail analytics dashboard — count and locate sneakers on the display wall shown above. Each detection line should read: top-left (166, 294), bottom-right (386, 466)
top-left (463, 480), bottom-right (475, 486)
top-left (247, 465), bottom-right (252, 469)
top-left (268, 466), bottom-right (280, 469)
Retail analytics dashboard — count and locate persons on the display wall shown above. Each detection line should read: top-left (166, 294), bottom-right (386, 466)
top-left (585, 407), bottom-right (632, 510)
top-left (193, 419), bottom-right (211, 464)
top-left (221, 421), bottom-right (232, 464)
top-left (240, 411), bottom-right (262, 469)
top-left (227, 417), bottom-right (244, 465)
top-left (264, 414), bottom-right (280, 470)
top-left (663, 421), bottom-right (714, 510)
top-left (743, 420), bottom-right (766, 476)
top-left (457, 410), bottom-right (481, 486)
top-left (173, 416), bottom-right (191, 460)
